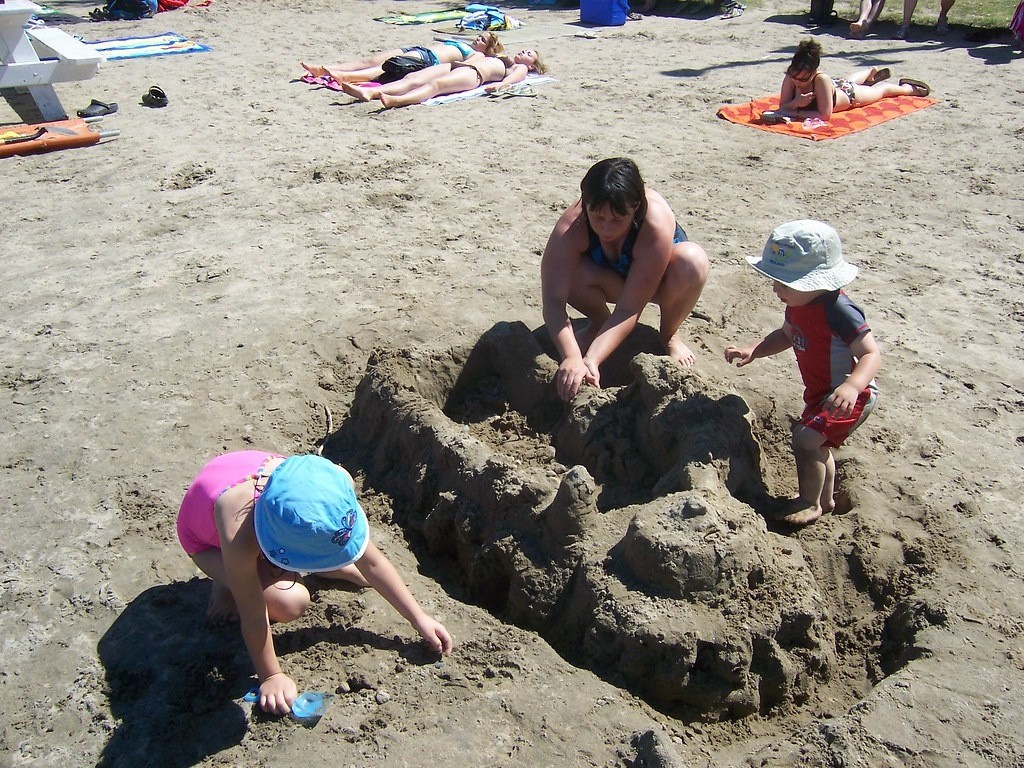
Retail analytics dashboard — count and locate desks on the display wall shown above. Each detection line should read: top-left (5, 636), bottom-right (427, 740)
top-left (0, 0), bottom-right (106, 122)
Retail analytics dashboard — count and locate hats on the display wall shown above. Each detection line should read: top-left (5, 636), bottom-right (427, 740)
top-left (254, 455), bottom-right (370, 572)
top-left (745, 220), bottom-right (859, 292)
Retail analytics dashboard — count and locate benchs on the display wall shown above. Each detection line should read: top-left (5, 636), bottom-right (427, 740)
top-left (25, 27), bottom-right (106, 65)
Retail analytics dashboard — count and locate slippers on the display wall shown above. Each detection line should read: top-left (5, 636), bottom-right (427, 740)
top-left (862, 67), bottom-right (890, 86)
top-left (720, 1), bottom-right (747, 18)
top-left (142, 86), bottom-right (168, 105)
top-left (77, 99), bottom-right (118, 118)
top-left (899, 79), bottom-right (930, 96)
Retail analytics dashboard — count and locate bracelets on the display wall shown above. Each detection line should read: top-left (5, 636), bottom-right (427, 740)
top-left (796, 110), bottom-right (800, 120)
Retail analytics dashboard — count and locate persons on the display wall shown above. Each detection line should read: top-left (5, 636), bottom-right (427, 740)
top-left (300, 31), bottom-right (505, 85)
top-left (342, 48), bottom-right (548, 108)
top-left (540, 157), bottom-right (709, 402)
top-left (779, 36), bottom-right (930, 121)
top-left (176, 450), bottom-right (453, 715)
top-left (104, 0), bottom-right (158, 21)
top-left (849, 0), bottom-right (955, 40)
top-left (724, 219), bottom-right (882, 524)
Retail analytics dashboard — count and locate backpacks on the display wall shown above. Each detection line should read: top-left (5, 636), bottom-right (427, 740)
top-left (382, 55), bottom-right (429, 77)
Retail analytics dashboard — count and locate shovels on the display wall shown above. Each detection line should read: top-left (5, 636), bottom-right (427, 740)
top-left (243, 686), bottom-right (336, 719)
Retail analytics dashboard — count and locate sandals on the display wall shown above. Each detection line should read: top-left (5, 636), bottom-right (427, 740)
top-left (807, 9), bottom-right (839, 28)
top-left (88, 7), bottom-right (120, 22)
top-left (896, 25), bottom-right (910, 39)
top-left (937, 17), bottom-right (948, 35)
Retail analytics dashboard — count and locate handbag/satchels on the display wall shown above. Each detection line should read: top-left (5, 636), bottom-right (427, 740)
top-left (580, 0), bottom-right (631, 26)
top-left (103, 0), bottom-right (189, 20)
